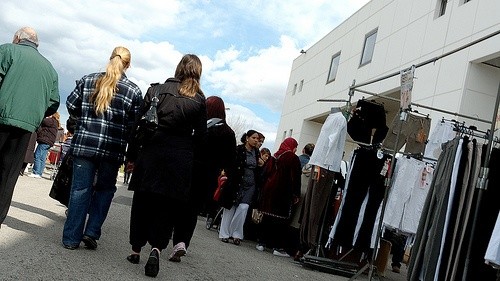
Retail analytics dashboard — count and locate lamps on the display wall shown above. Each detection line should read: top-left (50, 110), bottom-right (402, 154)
top-left (300, 50), bottom-right (306, 53)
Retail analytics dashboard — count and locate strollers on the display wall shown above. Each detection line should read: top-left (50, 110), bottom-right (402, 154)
top-left (205, 205), bottom-right (225, 235)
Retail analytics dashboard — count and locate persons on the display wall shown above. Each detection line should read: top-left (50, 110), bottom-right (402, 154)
top-left (0, 27), bottom-right (410, 279)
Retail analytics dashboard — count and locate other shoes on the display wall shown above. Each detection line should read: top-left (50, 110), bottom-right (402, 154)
top-left (82, 235), bottom-right (98, 249)
top-left (28, 173), bottom-right (42, 178)
top-left (127, 253), bottom-right (139, 263)
top-left (392, 266), bottom-right (400, 273)
top-left (273, 250), bottom-right (290, 257)
top-left (221, 238), bottom-right (229, 243)
top-left (256, 244), bottom-right (264, 251)
top-left (63, 242), bottom-right (78, 249)
top-left (234, 238), bottom-right (240, 246)
top-left (169, 242), bottom-right (187, 262)
top-left (145, 249), bottom-right (160, 277)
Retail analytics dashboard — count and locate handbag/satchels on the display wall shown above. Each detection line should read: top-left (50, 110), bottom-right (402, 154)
top-left (128, 83), bottom-right (160, 148)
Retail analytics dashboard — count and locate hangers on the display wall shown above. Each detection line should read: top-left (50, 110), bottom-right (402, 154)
top-left (400, 104), bottom-right (430, 120)
top-left (441, 113), bottom-right (500, 149)
top-left (362, 93), bottom-right (384, 106)
